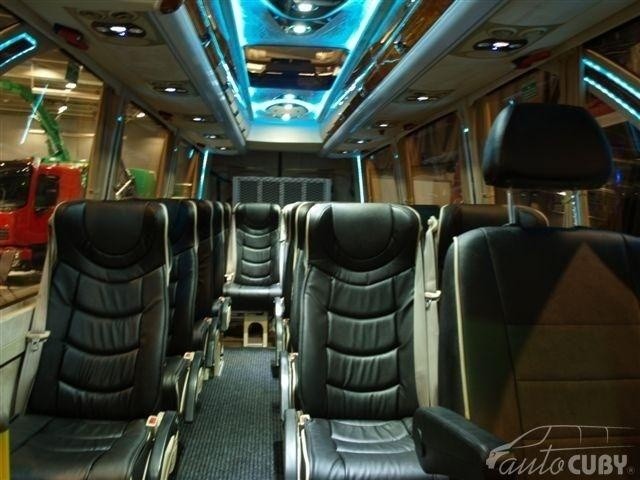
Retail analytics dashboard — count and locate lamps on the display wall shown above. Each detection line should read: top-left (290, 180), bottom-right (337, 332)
top-left (65, 61), bottom-right (79, 85)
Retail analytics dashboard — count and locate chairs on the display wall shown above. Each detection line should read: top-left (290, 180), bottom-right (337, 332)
top-left (9, 199), bottom-right (548, 480)
top-left (412, 100), bottom-right (640, 480)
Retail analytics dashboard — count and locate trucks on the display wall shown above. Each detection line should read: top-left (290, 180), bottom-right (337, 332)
top-left (0, 156), bottom-right (157, 271)
